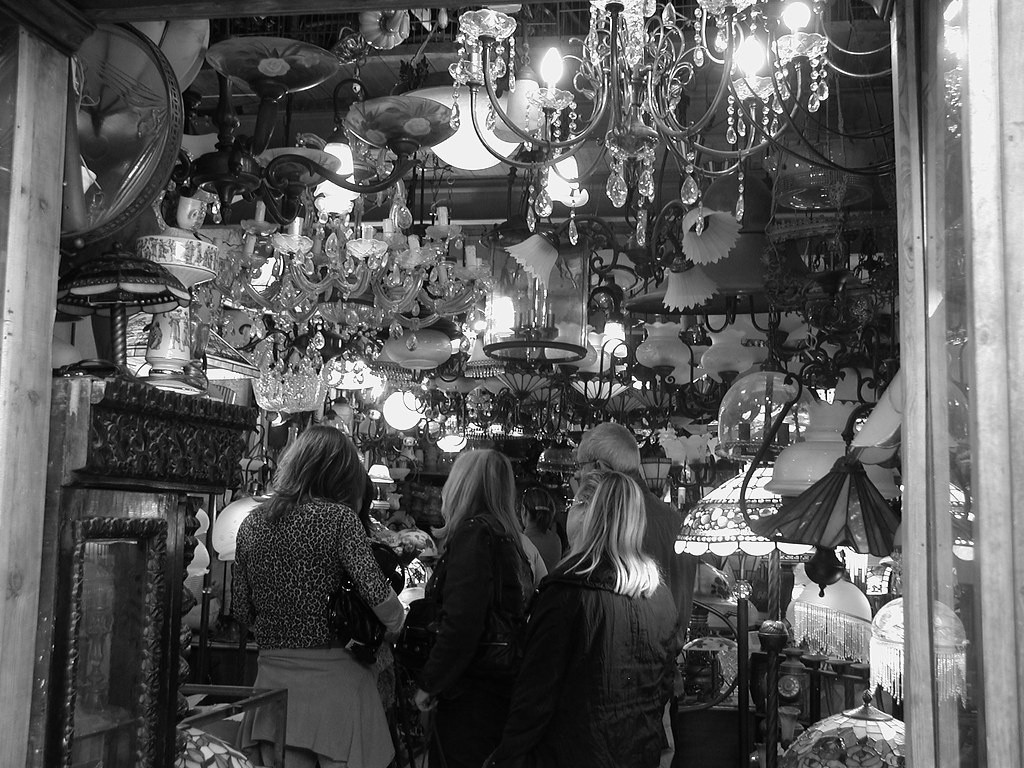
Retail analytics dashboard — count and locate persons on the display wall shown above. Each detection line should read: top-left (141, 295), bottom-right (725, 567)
top-left (232, 427), bottom-right (404, 767)
top-left (481, 472), bottom-right (677, 768)
top-left (414, 450), bottom-right (536, 767)
top-left (331, 424), bottom-right (696, 768)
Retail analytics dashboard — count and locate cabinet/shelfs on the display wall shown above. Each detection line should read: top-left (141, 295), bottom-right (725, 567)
top-left (23, 376), bottom-right (289, 768)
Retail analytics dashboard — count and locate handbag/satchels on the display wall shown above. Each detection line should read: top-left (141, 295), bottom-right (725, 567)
top-left (326, 542), bottom-right (405, 664)
top-left (395, 515), bottom-right (525, 681)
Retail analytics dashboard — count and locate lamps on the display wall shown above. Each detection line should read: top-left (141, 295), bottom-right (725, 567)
top-left (58, 0), bottom-right (970, 768)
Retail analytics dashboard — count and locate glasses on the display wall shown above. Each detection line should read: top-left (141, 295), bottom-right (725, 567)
top-left (573, 459), bottom-right (614, 471)
top-left (565, 498), bottom-right (591, 507)
